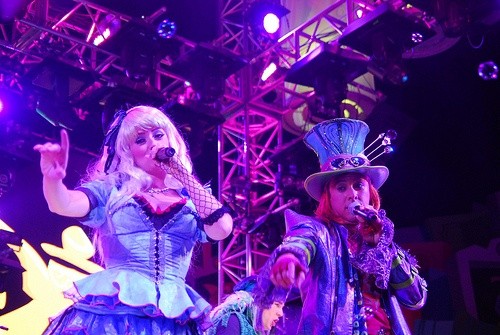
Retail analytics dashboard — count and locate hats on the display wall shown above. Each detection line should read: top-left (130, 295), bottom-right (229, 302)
top-left (303, 118), bottom-right (389, 203)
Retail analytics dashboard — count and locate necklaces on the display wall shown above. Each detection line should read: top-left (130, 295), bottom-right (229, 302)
top-left (143, 185), bottom-right (171, 194)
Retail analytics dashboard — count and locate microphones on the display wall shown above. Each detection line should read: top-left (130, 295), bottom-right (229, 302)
top-left (348, 200), bottom-right (377, 222)
top-left (155, 147), bottom-right (176, 161)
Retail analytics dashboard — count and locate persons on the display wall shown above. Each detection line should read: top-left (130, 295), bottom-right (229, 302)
top-left (210, 275), bottom-right (286, 335)
top-left (269, 117), bottom-right (428, 335)
top-left (33, 104), bottom-right (234, 335)
